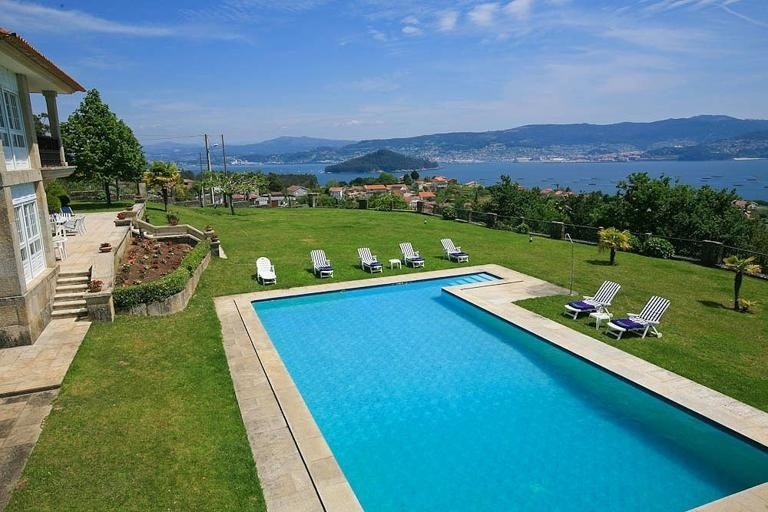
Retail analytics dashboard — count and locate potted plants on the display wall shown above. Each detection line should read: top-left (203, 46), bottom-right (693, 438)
top-left (211, 232), bottom-right (219, 242)
top-left (99, 243), bottom-right (112, 252)
top-left (125, 202), bottom-right (134, 211)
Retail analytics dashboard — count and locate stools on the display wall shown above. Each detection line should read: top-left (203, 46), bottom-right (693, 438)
top-left (389, 260), bottom-right (402, 271)
top-left (589, 311), bottom-right (610, 331)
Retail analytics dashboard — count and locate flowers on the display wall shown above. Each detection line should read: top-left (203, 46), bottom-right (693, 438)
top-left (88, 280), bottom-right (104, 288)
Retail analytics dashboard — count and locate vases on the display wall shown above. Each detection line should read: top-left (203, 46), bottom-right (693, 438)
top-left (91, 286), bottom-right (101, 292)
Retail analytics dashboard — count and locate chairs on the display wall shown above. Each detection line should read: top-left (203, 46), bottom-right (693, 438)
top-left (398, 242), bottom-right (426, 269)
top-left (604, 295), bottom-right (671, 341)
top-left (310, 249), bottom-right (334, 279)
top-left (48, 204), bottom-right (88, 262)
top-left (256, 256), bottom-right (277, 286)
top-left (357, 247), bottom-right (383, 275)
top-left (563, 280), bottom-right (622, 320)
top-left (440, 238), bottom-right (470, 264)
